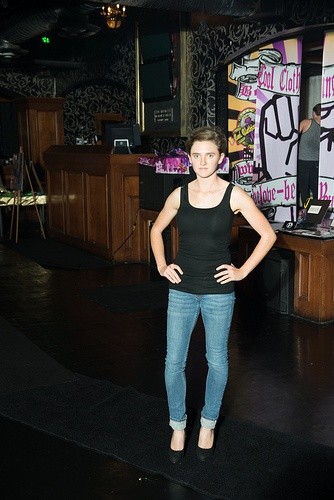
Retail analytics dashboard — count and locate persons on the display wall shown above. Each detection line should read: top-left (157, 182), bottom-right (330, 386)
top-left (298, 104), bottom-right (321, 208)
top-left (150, 127), bottom-right (276, 462)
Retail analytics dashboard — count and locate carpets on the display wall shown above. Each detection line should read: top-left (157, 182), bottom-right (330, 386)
top-left (1, 209), bottom-right (116, 271)
top-left (83, 281), bottom-right (167, 316)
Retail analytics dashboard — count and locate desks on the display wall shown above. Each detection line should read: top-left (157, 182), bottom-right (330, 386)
top-left (0, 190), bottom-right (47, 243)
top-left (238, 222), bottom-right (334, 325)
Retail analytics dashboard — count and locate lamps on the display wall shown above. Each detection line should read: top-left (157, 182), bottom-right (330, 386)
top-left (101, 2), bottom-right (128, 30)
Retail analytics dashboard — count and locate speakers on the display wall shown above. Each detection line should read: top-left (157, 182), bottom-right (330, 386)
top-left (25, 226), bottom-right (114, 270)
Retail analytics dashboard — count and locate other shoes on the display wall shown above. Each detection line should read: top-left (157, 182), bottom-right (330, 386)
top-left (168, 434), bottom-right (185, 464)
top-left (196, 432), bottom-right (216, 462)
